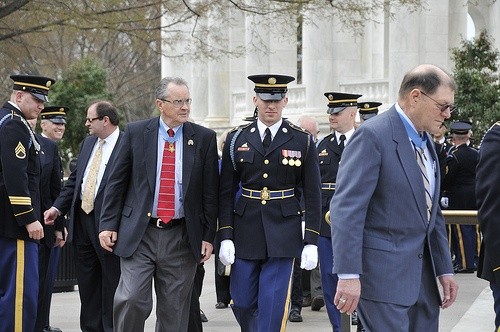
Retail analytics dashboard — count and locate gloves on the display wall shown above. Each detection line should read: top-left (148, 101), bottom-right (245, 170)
top-left (219, 241), bottom-right (236, 268)
top-left (441, 197), bottom-right (449, 208)
top-left (301, 245), bottom-right (318, 270)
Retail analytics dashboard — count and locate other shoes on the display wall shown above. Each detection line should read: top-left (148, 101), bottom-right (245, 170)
top-left (303, 297), bottom-right (311, 307)
top-left (289, 308), bottom-right (303, 322)
top-left (215, 302), bottom-right (228, 309)
top-left (311, 295), bottom-right (325, 311)
top-left (44, 328), bottom-right (62, 332)
top-left (452, 264), bottom-right (475, 273)
top-left (351, 312), bottom-right (358, 325)
top-left (200, 310), bottom-right (208, 322)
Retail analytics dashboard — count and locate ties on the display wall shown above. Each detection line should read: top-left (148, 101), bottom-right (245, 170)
top-left (415, 144), bottom-right (433, 227)
top-left (81, 140), bottom-right (106, 214)
top-left (157, 129), bottom-right (176, 223)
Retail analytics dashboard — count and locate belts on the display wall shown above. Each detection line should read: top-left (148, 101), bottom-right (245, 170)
top-left (149, 216), bottom-right (185, 227)
top-left (321, 183), bottom-right (337, 190)
top-left (239, 186), bottom-right (295, 202)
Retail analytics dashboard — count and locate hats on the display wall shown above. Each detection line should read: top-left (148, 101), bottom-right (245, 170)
top-left (357, 101), bottom-right (383, 119)
top-left (248, 74), bottom-right (296, 100)
top-left (324, 91), bottom-right (363, 114)
top-left (451, 123), bottom-right (472, 135)
top-left (10, 75), bottom-right (56, 103)
top-left (41, 106), bottom-right (70, 124)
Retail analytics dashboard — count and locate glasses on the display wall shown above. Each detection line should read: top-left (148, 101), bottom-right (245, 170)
top-left (86, 117), bottom-right (99, 122)
top-left (418, 91), bottom-right (457, 113)
top-left (157, 98), bottom-right (192, 107)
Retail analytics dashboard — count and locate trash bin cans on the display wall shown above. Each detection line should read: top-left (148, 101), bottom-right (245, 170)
top-left (52, 177), bottom-right (78, 293)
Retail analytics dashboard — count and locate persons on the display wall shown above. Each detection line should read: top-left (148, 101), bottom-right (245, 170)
top-left (188, 92), bottom-right (483, 332)
top-left (98, 76), bottom-right (220, 332)
top-left (474, 120), bottom-right (500, 332)
top-left (217, 79), bottom-right (322, 332)
top-left (329, 64), bottom-right (459, 332)
top-left (0, 75), bottom-right (68, 332)
top-left (43, 99), bottom-right (127, 332)
